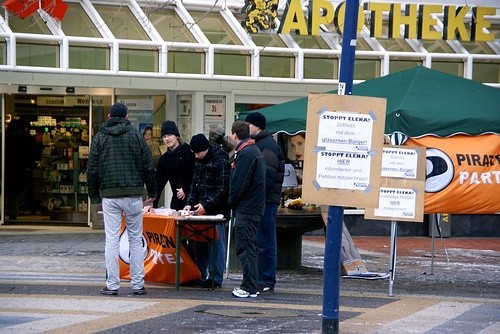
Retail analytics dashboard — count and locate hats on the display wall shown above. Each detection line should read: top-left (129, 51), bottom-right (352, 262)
top-left (110, 103), bottom-right (127, 118)
top-left (190, 134), bottom-right (209, 154)
top-left (245, 112), bottom-right (267, 130)
top-left (161, 121), bottom-right (180, 137)
top-left (209, 127), bottom-right (226, 143)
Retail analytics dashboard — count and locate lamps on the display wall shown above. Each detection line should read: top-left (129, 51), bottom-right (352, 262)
top-left (281, 164), bottom-right (299, 211)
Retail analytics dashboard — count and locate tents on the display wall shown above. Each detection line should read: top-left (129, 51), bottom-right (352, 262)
top-left (235, 64), bottom-right (499, 297)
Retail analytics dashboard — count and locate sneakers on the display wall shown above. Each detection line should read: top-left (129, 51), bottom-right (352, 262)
top-left (131, 287), bottom-right (148, 294)
top-left (100, 286), bottom-right (118, 295)
top-left (232, 287), bottom-right (260, 298)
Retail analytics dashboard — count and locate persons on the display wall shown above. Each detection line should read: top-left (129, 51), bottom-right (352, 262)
top-left (143, 121), bottom-right (195, 215)
top-left (228, 119), bottom-right (260, 299)
top-left (243, 111), bottom-right (284, 295)
top-left (182, 132), bottom-right (232, 290)
top-left (86, 103), bottom-right (158, 297)
top-left (6, 118), bottom-right (44, 221)
top-left (142, 125), bottom-right (152, 144)
top-left (288, 131), bottom-right (307, 182)
top-left (207, 126), bottom-right (238, 163)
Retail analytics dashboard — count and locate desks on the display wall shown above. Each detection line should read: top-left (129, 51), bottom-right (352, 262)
top-left (277, 206), bottom-right (324, 271)
top-left (97, 207), bottom-right (226, 294)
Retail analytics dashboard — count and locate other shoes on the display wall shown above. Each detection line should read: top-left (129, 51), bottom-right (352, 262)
top-left (202, 279), bottom-right (222, 288)
